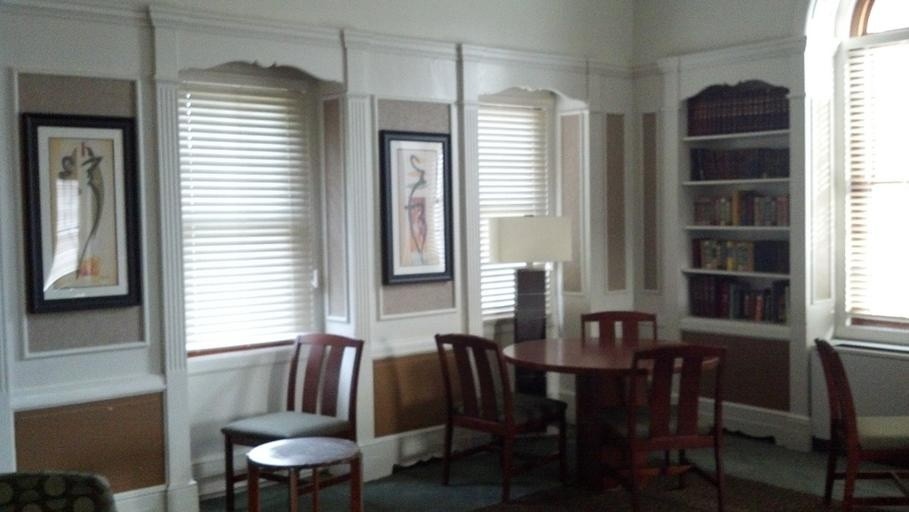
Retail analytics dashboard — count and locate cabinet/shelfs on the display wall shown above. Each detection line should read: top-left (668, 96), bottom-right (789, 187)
top-left (657, 32), bottom-right (803, 446)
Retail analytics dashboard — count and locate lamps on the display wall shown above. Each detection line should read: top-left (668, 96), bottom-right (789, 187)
top-left (488, 214), bottom-right (573, 433)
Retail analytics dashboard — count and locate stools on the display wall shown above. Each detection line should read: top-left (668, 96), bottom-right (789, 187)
top-left (245, 436), bottom-right (363, 512)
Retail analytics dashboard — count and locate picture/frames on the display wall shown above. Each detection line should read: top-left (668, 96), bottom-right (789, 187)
top-left (18, 110), bottom-right (144, 313)
top-left (378, 129), bottom-right (456, 285)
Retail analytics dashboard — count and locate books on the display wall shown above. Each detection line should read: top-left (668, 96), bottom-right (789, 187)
top-left (691, 237), bottom-right (788, 274)
top-left (688, 274), bottom-right (788, 322)
top-left (690, 146), bottom-right (789, 179)
top-left (687, 89), bottom-right (790, 138)
top-left (694, 190), bottom-right (790, 225)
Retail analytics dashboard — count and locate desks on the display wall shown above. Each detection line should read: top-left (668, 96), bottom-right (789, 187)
top-left (500, 337), bottom-right (719, 497)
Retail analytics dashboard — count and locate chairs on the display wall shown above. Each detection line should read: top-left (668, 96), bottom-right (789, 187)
top-left (220, 332), bottom-right (364, 512)
top-left (595, 343), bottom-right (727, 511)
top-left (812, 337), bottom-right (909, 512)
top-left (579, 311), bottom-right (658, 343)
top-left (433, 333), bottom-right (569, 503)
